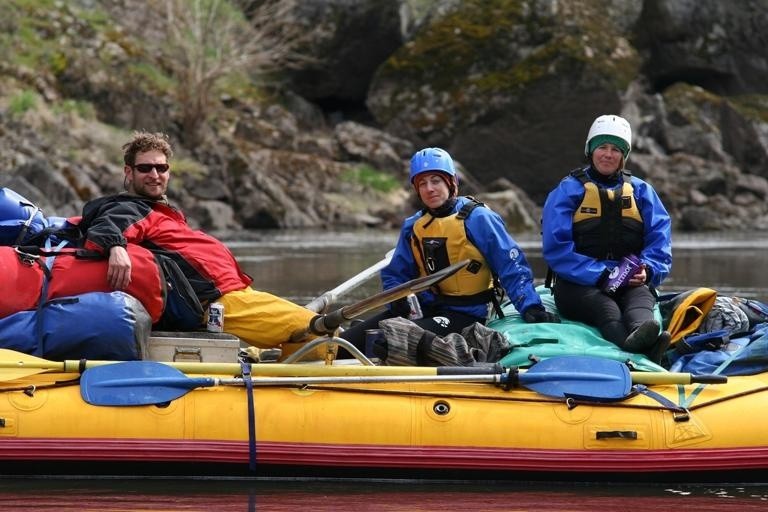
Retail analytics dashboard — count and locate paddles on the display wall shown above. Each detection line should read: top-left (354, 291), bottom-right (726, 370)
top-left (79, 357), bottom-right (631, 406)
top-left (287, 260), bottom-right (470, 343)
top-left (0, 348), bottom-right (728, 384)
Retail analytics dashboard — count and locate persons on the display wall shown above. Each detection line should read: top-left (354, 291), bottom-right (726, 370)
top-left (335, 146), bottom-right (562, 360)
top-left (82, 131), bottom-right (341, 363)
top-left (539, 113), bottom-right (673, 366)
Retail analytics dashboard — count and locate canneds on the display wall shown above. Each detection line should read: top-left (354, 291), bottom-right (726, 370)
top-left (206, 301), bottom-right (224, 333)
top-left (406, 293), bottom-right (423, 321)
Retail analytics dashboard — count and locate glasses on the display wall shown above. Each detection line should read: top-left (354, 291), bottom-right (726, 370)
top-left (132, 163), bottom-right (169, 173)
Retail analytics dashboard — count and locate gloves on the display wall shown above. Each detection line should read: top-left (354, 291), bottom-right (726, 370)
top-left (525, 304), bottom-right (562, 323)
top-left (391, 296), bottom-right (411, 319)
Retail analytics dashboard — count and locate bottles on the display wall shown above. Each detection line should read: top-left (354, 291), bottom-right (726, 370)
top-left (600, 254), bottom-right (640, 299)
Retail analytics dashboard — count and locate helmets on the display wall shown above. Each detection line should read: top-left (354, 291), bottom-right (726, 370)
top-left (409, 148), bottom-right (455, 184)
top-left (585, 114), bottom-right (633, 161)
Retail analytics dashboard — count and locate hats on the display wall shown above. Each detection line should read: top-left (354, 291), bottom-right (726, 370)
top-left (413, 171), bottom-right (451, 193)
top-left (590, 135), bottom-right (628, 159)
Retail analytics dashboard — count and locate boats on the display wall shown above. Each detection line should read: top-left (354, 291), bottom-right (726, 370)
top-left (0, 317), bottom-right (766, 474)
